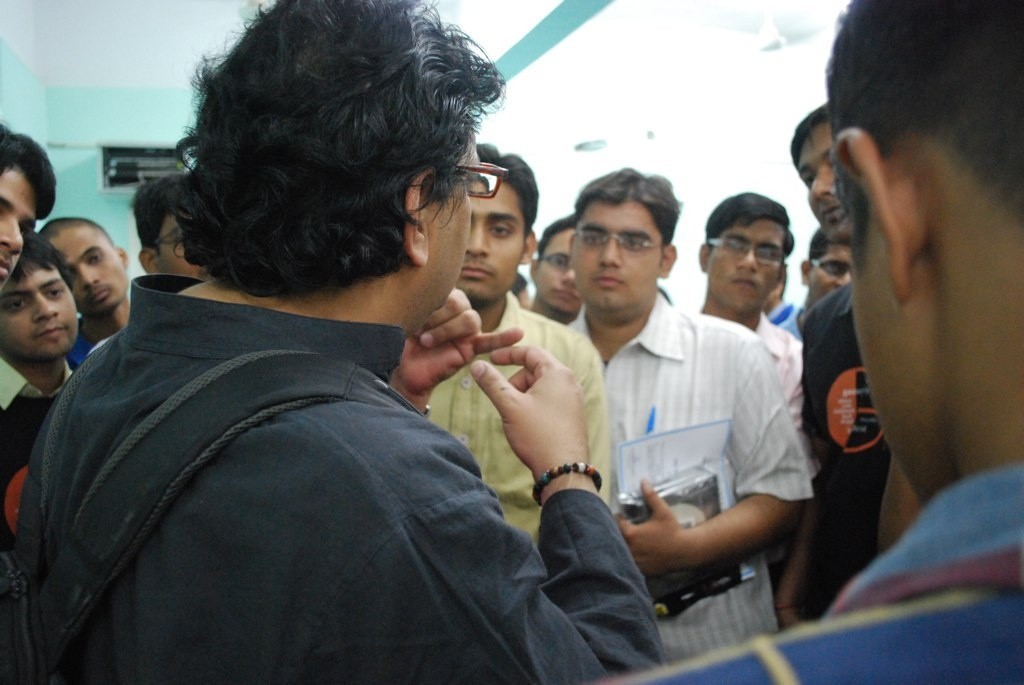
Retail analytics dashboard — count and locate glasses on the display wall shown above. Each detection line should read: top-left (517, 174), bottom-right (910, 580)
top-left (449, 162), bottom-right (508, 198)
top-left (707, 238), bottom-right (788, 266)
top-left (812, 261), bottom-right (849, 277)
top-left (572, 231), bottom-right (664, 250)
top-left (539, 252), bottom-right (568, 271)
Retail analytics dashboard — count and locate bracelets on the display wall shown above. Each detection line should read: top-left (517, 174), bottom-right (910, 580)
top-left (533, 462), bottom-right (603, 507)
top-left (776, 603), bottom-right (797, 610)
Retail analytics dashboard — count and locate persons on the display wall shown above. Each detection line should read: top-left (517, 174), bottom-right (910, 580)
top-left (775, 102), bottom-right (924, 631)
top-left (530, 215), bottom-right (590, 337)
top-left (15, 1), bottom-right (665, 683)
top-left (583, 0), bottom-right (1024, 685)
top-left (133, 174), bottom-right (216, 281)
top-left (567, 167), bottom-right (814, 666)
top-left (1, 123), bottom-right (129, 556)
top-left (697, 192), bottom-right (852, 344)
top-left (429, 144), bottom-right (612, 548)
